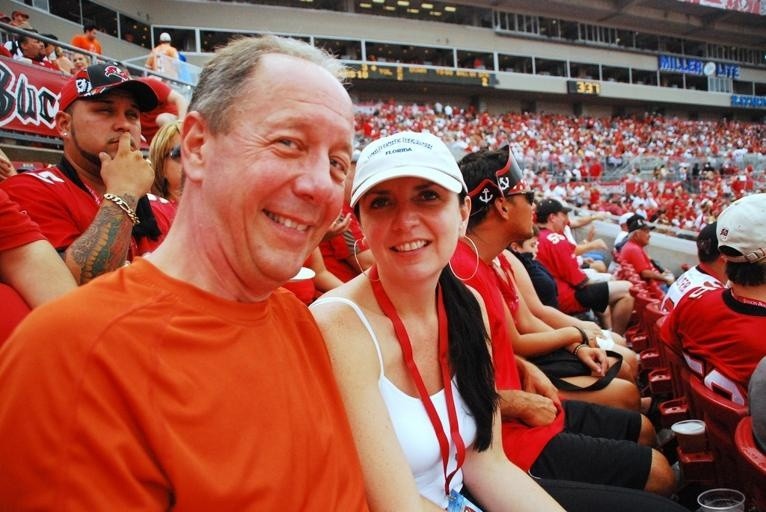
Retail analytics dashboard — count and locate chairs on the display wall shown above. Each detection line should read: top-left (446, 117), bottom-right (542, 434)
top-left (610, 248), bottom-right (766, 511)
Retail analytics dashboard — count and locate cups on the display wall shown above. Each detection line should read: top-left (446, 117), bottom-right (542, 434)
top-left (672, 418), bottom-right (709, 453)
top-left (697, 487), bottom-right (747, 512)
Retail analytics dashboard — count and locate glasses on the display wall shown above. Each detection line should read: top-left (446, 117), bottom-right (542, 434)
top-left (505, 191), bottom-right (536, 206)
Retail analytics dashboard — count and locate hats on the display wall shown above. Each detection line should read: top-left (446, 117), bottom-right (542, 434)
top-left (59, 62), bottom-right (158, 114)
top-left (160, 32), bottom-right (171, 42)
top-left (619, 212), bottom-right (655, 233)
top-left (716, 191), bottom-right (765, 264)
top-left (349, 130), bottom-right (470, 207)
top-left (11, 11), bottom-right (29, 20)
top-left (535, 198), bottom-right (572, 220)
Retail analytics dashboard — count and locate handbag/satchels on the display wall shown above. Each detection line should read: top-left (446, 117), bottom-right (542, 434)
top-left (525, 346), bottom-right (592, 377)
